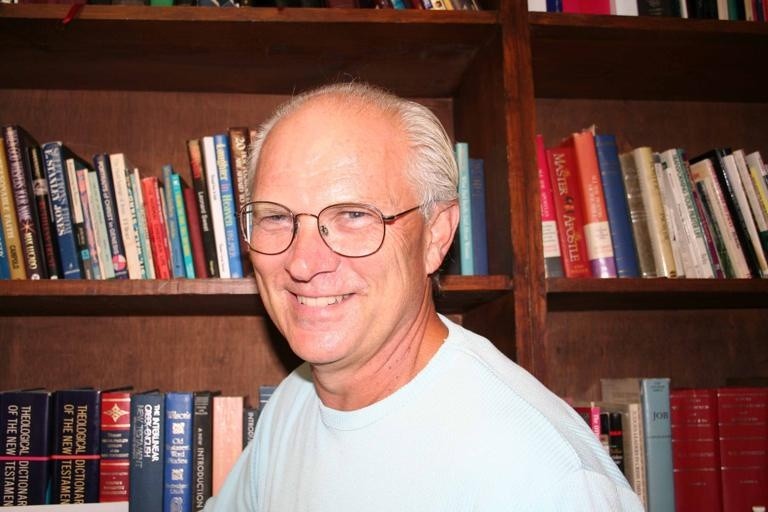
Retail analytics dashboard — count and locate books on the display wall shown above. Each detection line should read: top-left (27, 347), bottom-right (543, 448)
top-left (0, 385), bottom-right (278, 511)
top-left (0, 117), bottom-right (258, 281)
top-left (559, 376), bottom-right (768, 511)
top-left (436, 128), bottom-right (768, 284)
top-left (1, 0), bottom-right (768, 27)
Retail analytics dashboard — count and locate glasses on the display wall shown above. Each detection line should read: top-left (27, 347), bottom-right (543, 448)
top-left (236, 201), bottom-right (425, 261)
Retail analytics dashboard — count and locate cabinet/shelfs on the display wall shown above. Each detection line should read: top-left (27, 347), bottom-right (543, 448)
top-left (0, 0), bottom-right (768, 511)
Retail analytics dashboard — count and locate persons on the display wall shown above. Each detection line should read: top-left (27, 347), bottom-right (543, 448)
top-left (197, 80), bottom-right (646, 512)
